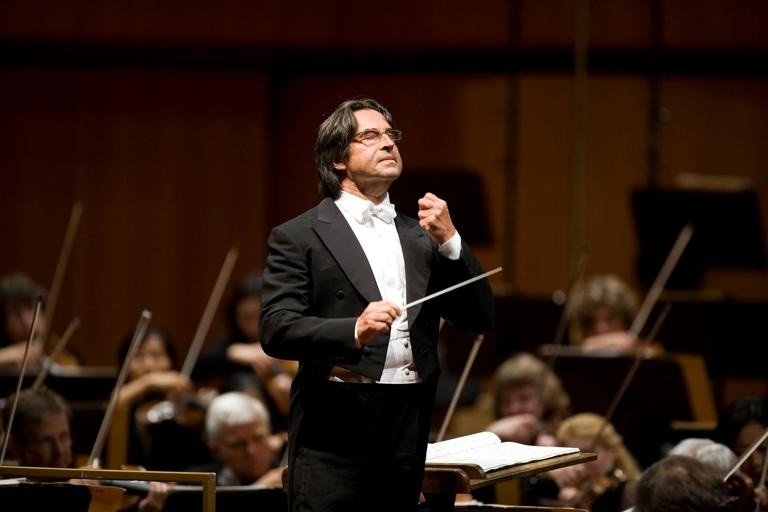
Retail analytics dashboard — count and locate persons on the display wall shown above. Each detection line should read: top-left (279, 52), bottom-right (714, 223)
top-left (634, 393), bottom-right (768, 512)
top-left (494, 273), bottom-right (682, 511)
top-left (1, 273), bottom-right (298, 511)
top-left (257, 98), bottom-right (495, 511)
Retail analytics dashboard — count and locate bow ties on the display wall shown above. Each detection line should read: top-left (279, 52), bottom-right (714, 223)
top-left (347, 199), bottom-right (397, 224)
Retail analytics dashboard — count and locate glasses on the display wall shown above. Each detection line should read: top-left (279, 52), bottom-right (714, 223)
top-left (353, 130), bottom-right (402, 140)
